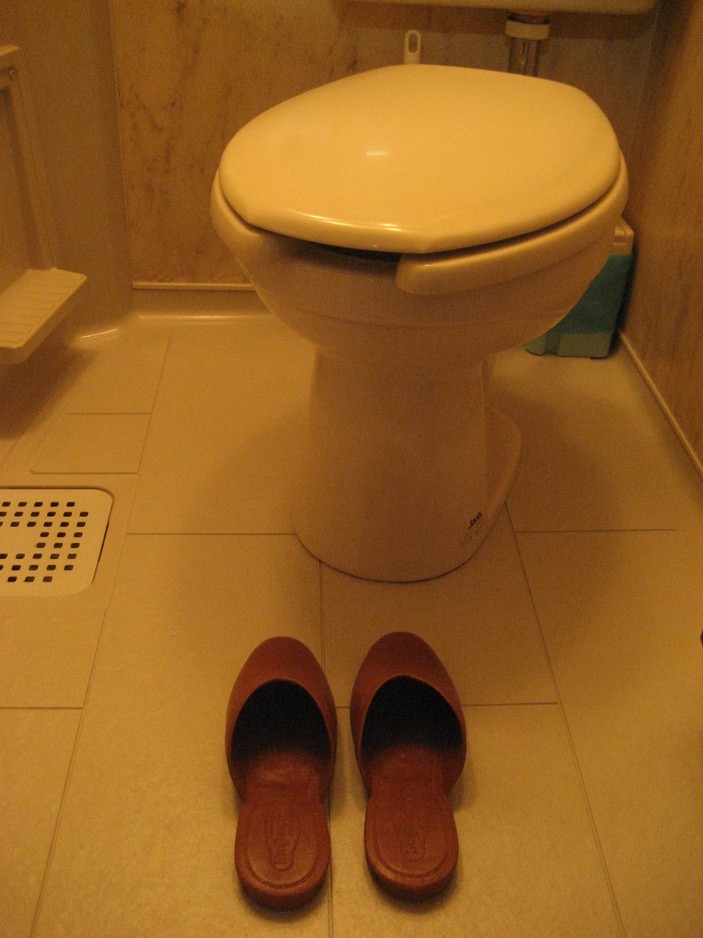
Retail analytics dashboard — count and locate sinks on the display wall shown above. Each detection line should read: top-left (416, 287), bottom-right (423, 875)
top-left (384, 0), bottom-right (655, 16)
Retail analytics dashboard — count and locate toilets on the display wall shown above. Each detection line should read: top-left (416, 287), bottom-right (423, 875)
top-left (209, 62), bottom-right (633, 589)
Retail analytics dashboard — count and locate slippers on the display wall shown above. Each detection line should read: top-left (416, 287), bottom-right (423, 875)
top-left (224, 637), bottom-right (339, 909)
top-left (351, 630), bottom-right (468, 901)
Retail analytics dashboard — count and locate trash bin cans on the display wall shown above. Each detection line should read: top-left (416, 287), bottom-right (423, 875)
top-left (525, 216), bottom-right (635, 359)
